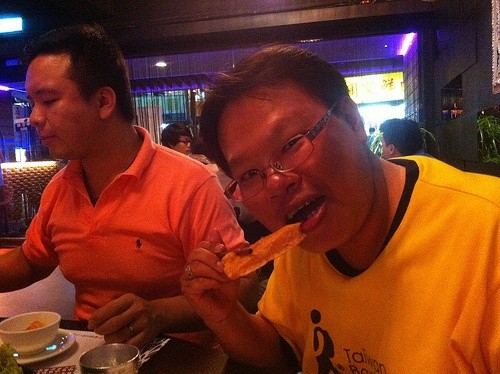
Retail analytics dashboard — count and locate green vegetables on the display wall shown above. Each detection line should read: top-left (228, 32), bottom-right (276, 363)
top-left (0, 341), bottom-right (25, 374)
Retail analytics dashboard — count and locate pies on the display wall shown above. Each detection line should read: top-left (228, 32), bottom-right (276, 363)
top-left (221, 221), bottom-right (306, 280)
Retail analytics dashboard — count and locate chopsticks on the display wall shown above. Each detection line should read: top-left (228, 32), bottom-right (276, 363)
top-left (216, 248), bottom-right (259, 280)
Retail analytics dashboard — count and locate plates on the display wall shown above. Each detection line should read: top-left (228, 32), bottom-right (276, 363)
top-left (0, 328), bottom-right (76, 364)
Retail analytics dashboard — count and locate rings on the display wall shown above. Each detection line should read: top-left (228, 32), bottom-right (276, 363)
top-left (186, 266), bottom-right (194, 279)
top-left (128, 325), bottom-right (136, 336)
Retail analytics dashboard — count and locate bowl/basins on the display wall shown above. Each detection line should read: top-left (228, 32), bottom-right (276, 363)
top-left (0, 311), bottom-right (62, 355)
top-left (79, 343), bottom-right (140, 374)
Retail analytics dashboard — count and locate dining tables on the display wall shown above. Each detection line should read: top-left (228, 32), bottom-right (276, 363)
top-left (0, 317), bottom-right (230, 374)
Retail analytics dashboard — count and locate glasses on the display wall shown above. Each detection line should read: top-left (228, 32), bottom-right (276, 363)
top-left (173, 138), bottom-right (190, 145)
top-left (223, 99), bottom-right (339, 202)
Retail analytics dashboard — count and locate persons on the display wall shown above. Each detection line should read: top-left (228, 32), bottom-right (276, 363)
top-left (0, 27), bottom-right (259, 348)
top-left (161, 119), bottom-right (232, 186)
top-left (380, 120), bottom-right (434, 158)
top-left (180, 45), bottom-right (500, 374)
